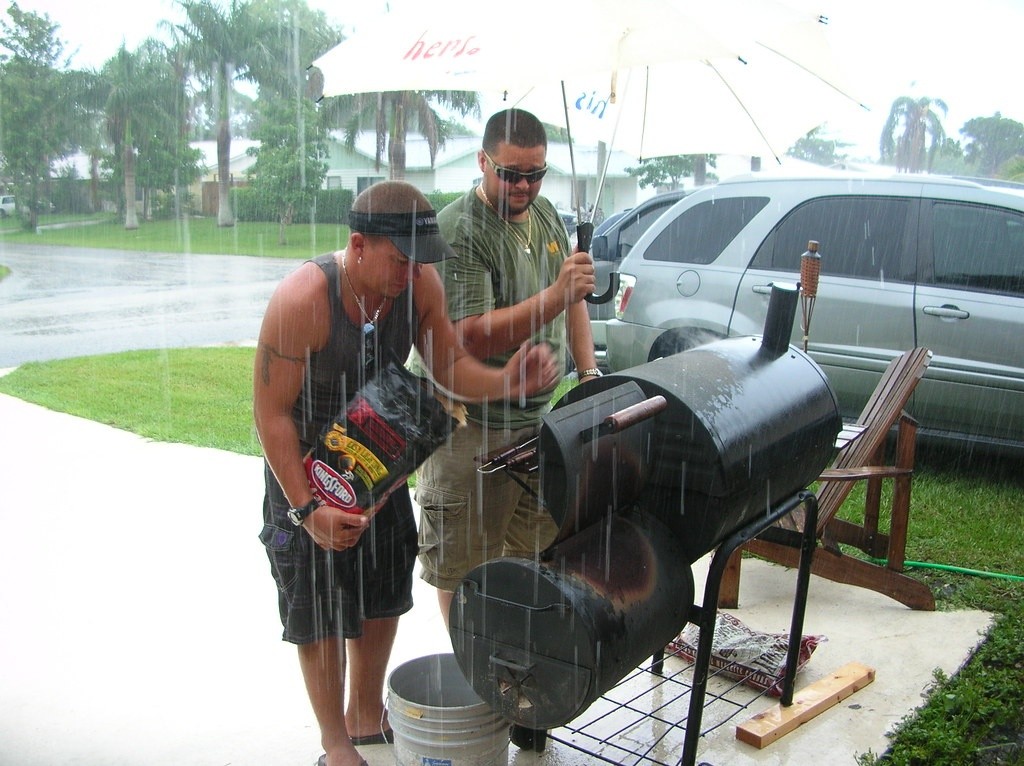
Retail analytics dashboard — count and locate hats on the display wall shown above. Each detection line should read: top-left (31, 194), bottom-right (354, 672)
top-left (346, 208), bottom-right (460, 265)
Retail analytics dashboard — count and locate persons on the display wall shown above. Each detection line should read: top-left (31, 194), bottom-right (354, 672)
top-left (252, 181), bottom-right (559, 766)
top-left (407, 107), bottom-right (604, 634)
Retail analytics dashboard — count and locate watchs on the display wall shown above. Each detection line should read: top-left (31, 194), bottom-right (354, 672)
top-left (287, 494), bottom-right (327, 526)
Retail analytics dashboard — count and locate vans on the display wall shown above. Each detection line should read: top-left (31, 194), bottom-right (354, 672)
top-left (607, 175), bottom-right (1024, 465)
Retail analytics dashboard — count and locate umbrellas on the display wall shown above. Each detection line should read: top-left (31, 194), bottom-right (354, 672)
top-left (311, 0), bottom-right (884, 304)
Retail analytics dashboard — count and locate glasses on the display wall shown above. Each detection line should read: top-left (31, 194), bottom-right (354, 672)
top-left (481, 148), bottom-right (549, 184)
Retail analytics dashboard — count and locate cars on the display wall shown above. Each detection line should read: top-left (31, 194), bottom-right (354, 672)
top-left (588, 208), bottom-right (631, 243)
top-left (556, 207), bottom-right (578, 237)
top-left (573, 186), bottom-right (698, 354)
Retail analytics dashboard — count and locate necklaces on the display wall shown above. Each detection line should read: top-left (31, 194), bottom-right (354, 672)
top-left (342, 259), bottom-right (386, 334)
top-left (480, 181), bottom-right (531, 254)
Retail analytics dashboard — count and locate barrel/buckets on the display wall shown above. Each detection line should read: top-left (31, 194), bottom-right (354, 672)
top-left (381, 654), bottom-right (516, 766)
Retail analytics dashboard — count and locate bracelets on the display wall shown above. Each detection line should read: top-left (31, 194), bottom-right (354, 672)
top-left (578, 368), bottom-right (603, 383)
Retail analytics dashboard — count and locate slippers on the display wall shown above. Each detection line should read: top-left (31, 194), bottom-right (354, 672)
top-left (349, 728), bottom-right (395, 746)
top-left (318, 754), bottom-right (370, 766)
